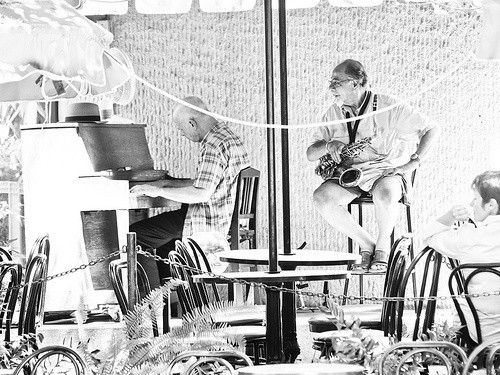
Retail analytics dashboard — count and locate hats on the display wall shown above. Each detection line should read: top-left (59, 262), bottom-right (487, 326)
top-left (65, 102), bottom-right (100, 122)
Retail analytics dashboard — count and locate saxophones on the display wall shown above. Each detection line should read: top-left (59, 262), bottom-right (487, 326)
top-left (313, 134), bottom-right (379, 189)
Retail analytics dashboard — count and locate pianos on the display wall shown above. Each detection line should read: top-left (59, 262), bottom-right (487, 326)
top-left (19, 121), bottom-right (197, 313)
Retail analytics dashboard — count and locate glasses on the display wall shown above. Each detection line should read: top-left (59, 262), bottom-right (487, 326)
top-left (328, 78), bottom-right (355, 88)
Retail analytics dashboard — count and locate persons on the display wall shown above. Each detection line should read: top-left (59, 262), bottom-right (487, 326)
top-left (428, 172), bottom-right (500, 347)
top-left (126, 97), bottom-right (251, 326)
top-left (306, 59), bottom-right (443, 276)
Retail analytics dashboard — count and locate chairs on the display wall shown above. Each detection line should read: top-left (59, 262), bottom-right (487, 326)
top-left (227, 166), bottom-right (261, 305)
top-left (312, 235), bottom-right (500, 375)
top-left (0, 232), bottom-right (221, 375)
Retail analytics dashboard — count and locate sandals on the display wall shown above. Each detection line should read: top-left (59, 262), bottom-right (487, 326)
top-left (351, 250), bottom-right (373, 272)
top-left (368, 249), bottom-right (389, 273)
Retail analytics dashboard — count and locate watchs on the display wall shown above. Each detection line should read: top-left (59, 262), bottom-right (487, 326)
top-left (410, 153), bottom-right (422, 166)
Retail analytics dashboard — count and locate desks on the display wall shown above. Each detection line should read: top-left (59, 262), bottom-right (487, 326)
top-left (237, 363), bottom-right (368, 375)
top-left (192, 269), bottom-right (346, 284)
top-left (218, 249), bottom-right (369, 267)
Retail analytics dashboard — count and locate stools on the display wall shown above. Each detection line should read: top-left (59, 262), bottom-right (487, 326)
top-left (342, 193), bottom-right (418, 312)
